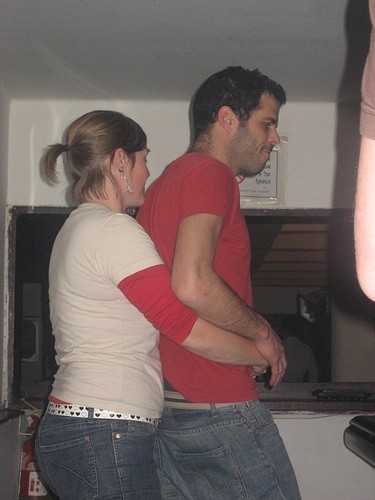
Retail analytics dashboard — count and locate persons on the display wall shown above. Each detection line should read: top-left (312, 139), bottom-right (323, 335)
top-left (133, 64), bottom-right (302, 500)
top-left (353, 0), bottom-right (375, 305)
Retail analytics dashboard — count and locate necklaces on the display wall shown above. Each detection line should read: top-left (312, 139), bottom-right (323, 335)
top-left (34, 110), bottom-right (271, 499)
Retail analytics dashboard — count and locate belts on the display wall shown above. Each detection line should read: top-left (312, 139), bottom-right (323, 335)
top-left (164, 390), bottom-right (252, 410)
top-left (46, 400), bottom-right (160, 428)
